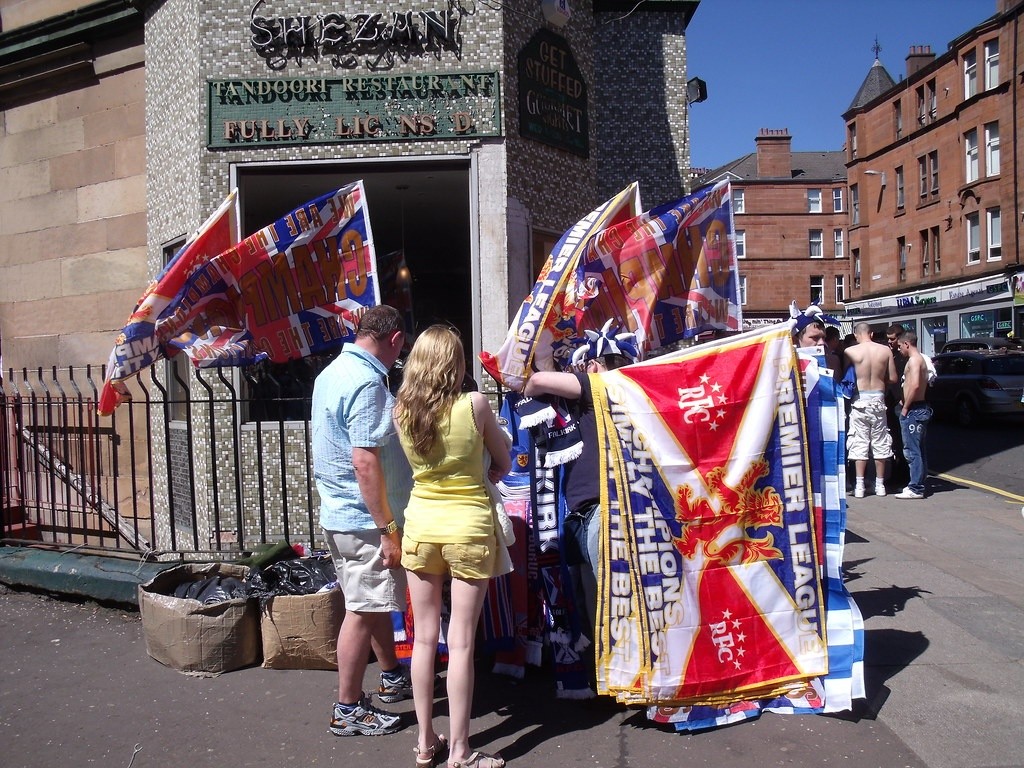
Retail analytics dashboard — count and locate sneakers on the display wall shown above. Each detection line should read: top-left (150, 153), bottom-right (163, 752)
top-left (329, 691), bottom-right (404, 736)
top-left (378, 662), bottom-right (441, 703)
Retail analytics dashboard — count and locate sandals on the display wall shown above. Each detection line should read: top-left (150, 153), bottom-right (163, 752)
top-left (447, 749), bottom-right (505, 768)
top-left (413, 733), bottom-right (448, 768)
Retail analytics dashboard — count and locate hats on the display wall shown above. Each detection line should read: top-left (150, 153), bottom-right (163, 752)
top-left (787, 298), bottom-right (841, 337)
top-left (567, 317), bottom-right (641, 366)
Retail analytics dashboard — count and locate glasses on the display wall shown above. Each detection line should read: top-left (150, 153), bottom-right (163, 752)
top-left (577, 359), bottom-right (604, 373)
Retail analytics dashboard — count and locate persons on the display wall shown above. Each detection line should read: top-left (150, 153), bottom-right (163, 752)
top-left (788, 299), bottom-right (938, 500)
top-left (310, 304), bottom-right (440, 738)
top-left (524, 318), bottom-right (639, 634)
top-left (392, 324), bottom-right (514, 768)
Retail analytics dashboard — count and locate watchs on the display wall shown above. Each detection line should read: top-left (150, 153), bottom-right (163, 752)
top-left (378, 518), bottom-right (398, 536)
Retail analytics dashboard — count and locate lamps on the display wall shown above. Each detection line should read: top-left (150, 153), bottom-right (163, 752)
top-left (393, 184), bottom-right (414, 287)
top-left (686, 76), bottom-right (708, 105)
top-left (864, 170), bottom-right (886, 186)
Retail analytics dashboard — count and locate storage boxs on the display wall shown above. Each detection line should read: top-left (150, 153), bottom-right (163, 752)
top-left (256, 553), bottom-right (373, 671)
top-left (138, 563), bottom-right (262, 679)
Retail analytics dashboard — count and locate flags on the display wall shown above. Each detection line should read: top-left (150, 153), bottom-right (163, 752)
top-left (155, 179), bottom-right (382, 369)
top-left (475, 175), bottom-right (743, 393)
top-left (94, 185), bottom-right (239, 418)
top-left (587, 318), bottom-right (868, 732)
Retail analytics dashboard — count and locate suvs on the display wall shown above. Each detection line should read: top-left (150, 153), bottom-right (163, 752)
top-left (923, 346), bottom-right (1024, 429)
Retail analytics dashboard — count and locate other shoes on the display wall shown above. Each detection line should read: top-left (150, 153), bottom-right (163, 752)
top-left (895, 490), bottom-right (924, 498)
top-left (852, 483), bottom-right (865, 497)
top-left (897, 486), bottom-right (909, 492)
top-left (867, 482), bottom-right (886, 496)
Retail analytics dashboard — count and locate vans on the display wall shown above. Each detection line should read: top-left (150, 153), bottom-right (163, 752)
top-left (938, 336), bottom-right (1018, 355)
